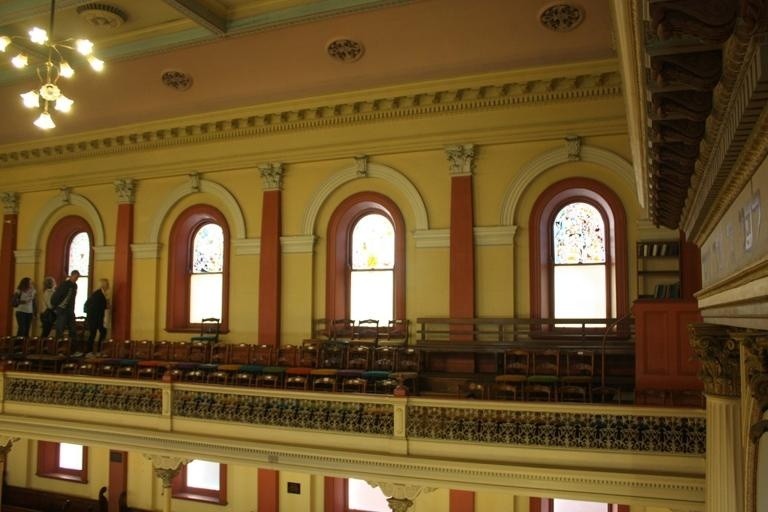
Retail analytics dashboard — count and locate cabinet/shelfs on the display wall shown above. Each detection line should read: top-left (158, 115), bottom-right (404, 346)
top-left (626, 227), bottom-right (705, 409)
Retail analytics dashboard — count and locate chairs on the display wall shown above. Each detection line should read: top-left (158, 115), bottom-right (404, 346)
top-left (0, 316), bottom-right (632, 403)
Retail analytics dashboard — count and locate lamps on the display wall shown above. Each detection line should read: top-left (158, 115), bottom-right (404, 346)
top-left (0, 0), bottom-right (112, 134)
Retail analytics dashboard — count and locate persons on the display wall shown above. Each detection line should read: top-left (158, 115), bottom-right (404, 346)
top-left (50, 271), bottom-right (84, 358)
top-left (84, 280), bottom-right (110, 358)
top-left (15, 277), bottom-right (37, 337)
top-left (38, 277), bottom-right (56, 338)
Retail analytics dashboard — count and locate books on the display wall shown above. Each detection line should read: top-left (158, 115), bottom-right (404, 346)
top-left (638, 242), bottom-right (677, 256)
top-left (653, 283), bottom-right (678, 297)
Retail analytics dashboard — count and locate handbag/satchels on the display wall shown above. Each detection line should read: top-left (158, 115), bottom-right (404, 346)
top-left (42, 308), bottom-right (56, 324)
